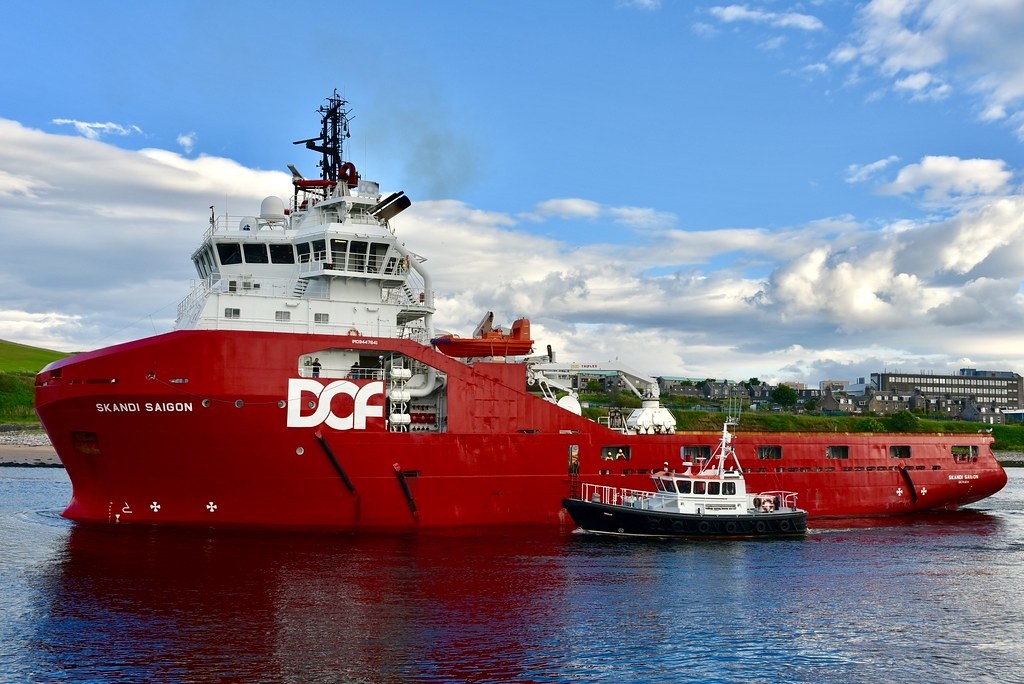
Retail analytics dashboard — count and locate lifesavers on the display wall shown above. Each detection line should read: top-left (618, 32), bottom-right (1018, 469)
top-left (348, 329), bottom-right (359, 336)
top-left (340, 162), bottom-right (356, 182)
top-left (406, 255), bottom-right (410, 268)
top-left (762, 498), bottom-right (773, 511)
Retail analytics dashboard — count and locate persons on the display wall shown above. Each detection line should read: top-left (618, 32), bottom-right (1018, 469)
top-left (350, 362), bottom-right (360, 378)
top-left (311, 358), bottom-right (322, 378)
top-left (615, 449), bottom-right (626, 460)
top-left (605, 452), bottom-right (613, 461)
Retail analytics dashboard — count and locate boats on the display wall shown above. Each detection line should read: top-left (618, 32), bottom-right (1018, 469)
top-left (34, 89), bottom-right (1008, 535)
top-left (561, 396), bottom-right (808, 535)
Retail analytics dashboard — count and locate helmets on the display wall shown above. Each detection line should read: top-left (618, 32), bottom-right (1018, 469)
top-left (618, 449), bottom-right (623, 453)
top-left (608, 451), bottom-right (612, 456)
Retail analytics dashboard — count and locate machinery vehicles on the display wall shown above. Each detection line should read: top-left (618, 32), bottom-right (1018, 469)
top-left (527, 363), bottom-right (676, 435)
